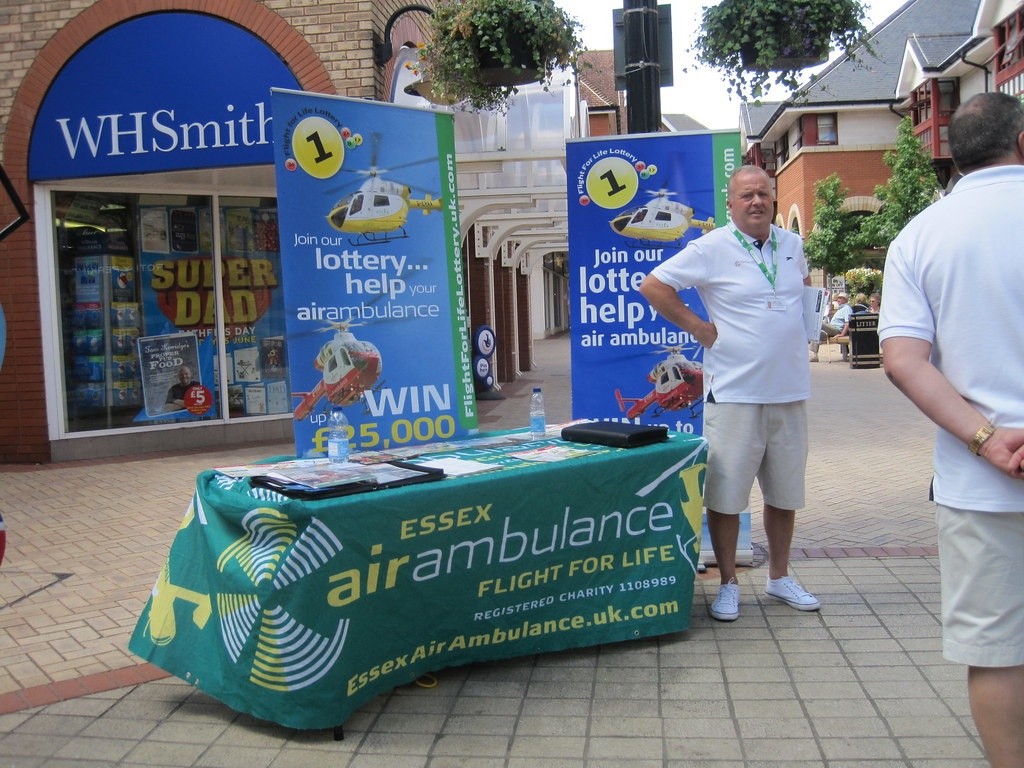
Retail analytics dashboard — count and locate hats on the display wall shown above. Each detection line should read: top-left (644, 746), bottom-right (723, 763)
top-left (838, 292), bottom-right (849, 300)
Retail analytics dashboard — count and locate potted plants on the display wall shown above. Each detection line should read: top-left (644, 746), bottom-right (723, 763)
top-left (405, 0), bottom-right (593, 124)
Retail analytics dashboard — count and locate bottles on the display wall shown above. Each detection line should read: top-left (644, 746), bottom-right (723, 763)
top-left (530, 388), bottom-right (546, 439)
top-left (328, 405), bottom-right (349, 463)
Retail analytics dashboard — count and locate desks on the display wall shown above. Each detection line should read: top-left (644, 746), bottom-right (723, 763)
top-left (196, 424), bottom-right (707, 741)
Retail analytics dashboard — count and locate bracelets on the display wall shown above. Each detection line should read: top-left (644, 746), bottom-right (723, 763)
top-left (967, 424), bottom-right (996, 456)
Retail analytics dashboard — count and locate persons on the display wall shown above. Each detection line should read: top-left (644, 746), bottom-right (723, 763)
top-left (877, 92), bottom-right (1024, 768)
top-left (865, 293), bottom-right (881, 313)
top-left (639, 165), bottom-right (821, 621)
top-left (851, 293), bottom-right (870, 314)
top-left (809, 292), bottom-right (853, 362)
top-left (165, 367), bottom-right (200, 412)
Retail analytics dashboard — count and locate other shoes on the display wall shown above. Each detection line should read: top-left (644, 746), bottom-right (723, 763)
top-left (809, 355), bottom-right (819, 362)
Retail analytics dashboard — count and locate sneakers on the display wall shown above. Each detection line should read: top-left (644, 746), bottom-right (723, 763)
top-left (764, 575), bottom-right (820, 610)
top-left (709, 577), bottom-right (739, 620)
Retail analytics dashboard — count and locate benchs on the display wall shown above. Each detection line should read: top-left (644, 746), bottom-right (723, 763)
top-left (823, 305), bottom-right (869, 362)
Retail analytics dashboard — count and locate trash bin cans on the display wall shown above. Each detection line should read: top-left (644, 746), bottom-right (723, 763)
top-left (848, 311), bottom-right (881, 369)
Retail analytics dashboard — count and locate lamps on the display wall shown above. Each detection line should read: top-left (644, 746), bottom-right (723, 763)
top-left (378, 4), bottom-right (470, 106)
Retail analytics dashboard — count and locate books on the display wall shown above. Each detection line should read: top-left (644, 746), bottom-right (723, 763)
top-left (415, 458), bottom-right (504, 477)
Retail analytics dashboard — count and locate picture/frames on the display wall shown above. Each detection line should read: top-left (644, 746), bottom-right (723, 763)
top-left (683, 0), bottom-right (888, 108)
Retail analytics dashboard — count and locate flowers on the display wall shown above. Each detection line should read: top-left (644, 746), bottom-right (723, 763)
top-left (845, 267), bottom-right (884, 295)
